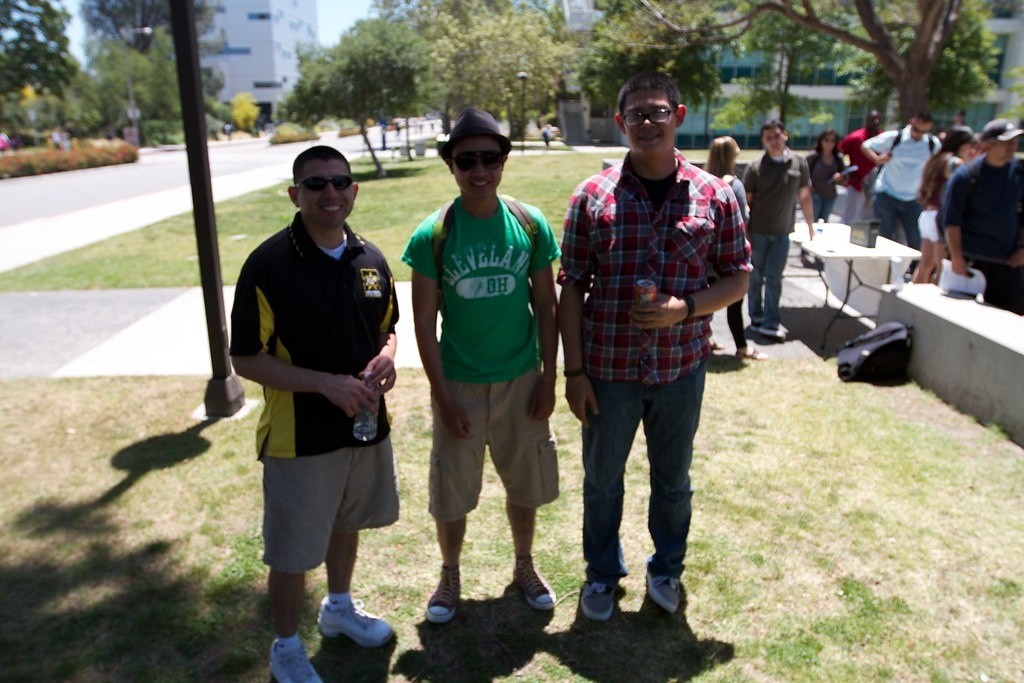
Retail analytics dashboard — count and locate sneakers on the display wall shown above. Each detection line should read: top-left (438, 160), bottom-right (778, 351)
top-left (270, 638), bottom-right (322, 683)
top-left (426, 563), bottom-right (460, 623)
top-left (581, 580), bottom-right (616, 621)
top-left (514, 556), bottom-right (556, 610)
top-left (646, 567), bottom-right (680, 614)
top-left (317, 599), bottom-right (393, 647)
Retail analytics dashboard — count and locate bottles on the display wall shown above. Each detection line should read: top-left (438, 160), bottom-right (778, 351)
top-left (353, 371), bottom-right (380, 442)
top-left (814, 218), bottom-right (825, 240)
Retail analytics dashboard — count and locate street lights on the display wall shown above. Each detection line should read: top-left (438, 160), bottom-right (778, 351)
top-left (704, 44), bottom-right (722, 149)
top-left (517, 71), bottom-right (528, 151)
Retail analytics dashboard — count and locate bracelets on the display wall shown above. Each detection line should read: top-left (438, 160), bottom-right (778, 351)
top-left (564, 369), bottom-right (586, 377)
top-left (682, 294), bottom-right (695, 323)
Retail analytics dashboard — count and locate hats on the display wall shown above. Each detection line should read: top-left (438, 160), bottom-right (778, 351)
top-left (981, 119), bottom-right (1024, 141)
top-left (440, 108), bottom-right (512, 160)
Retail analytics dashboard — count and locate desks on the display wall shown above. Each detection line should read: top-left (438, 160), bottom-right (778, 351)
top-left (789, 223), bottom-right (923, 350)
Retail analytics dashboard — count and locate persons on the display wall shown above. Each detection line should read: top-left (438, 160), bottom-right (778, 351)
top-left (229, 145), bottom-right (400, 682)
top-left (702, 136), bottom-right (767, 360)
top-left (800, 129), bottom-right (847, 266)
top-left (742, 119), bottom-right (817, 340)
top-left (944, 118), bottom-right (1024, 317)
top-left (560, 71), bottom-right (750, 620)
top-left (402, 108), bottom-right (559, 623)
top-left (835, 108), bottom-right (985, 284)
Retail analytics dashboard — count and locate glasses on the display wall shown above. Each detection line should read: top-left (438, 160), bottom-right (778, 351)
top-left (450, 150), bottom-right (505, 171)
top-left (621, 109), bottom-right (675, 126)
top-left (296, 175), bottom-right (353, 191)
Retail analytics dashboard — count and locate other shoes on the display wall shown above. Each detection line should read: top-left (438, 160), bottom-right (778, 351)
top-left (709, 341), bottom-right (724, 349)
top-left (759, 326), bottom-right (786, 341)
top-left (751, 322), bottom-right (762, 331)
top-left (736, 350), bottom-right (768, 360)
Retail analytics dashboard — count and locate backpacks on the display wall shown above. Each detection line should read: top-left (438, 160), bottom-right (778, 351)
top-left (836, 321), bottom-right (915, 386)
